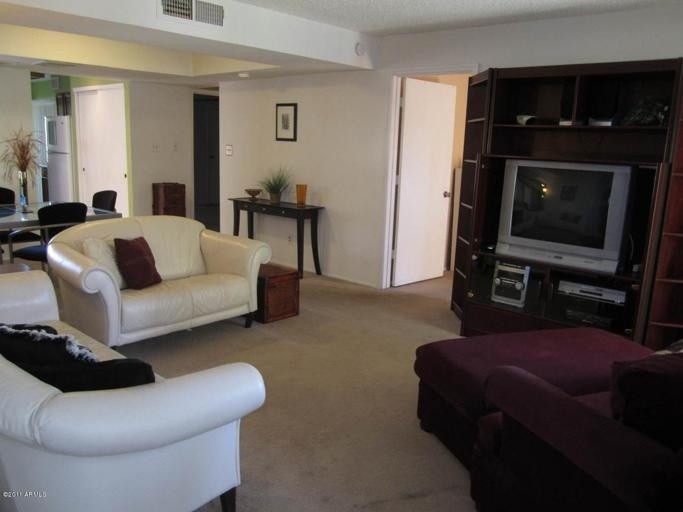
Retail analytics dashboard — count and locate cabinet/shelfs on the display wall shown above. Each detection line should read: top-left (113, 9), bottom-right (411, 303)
top-left (450, 58), bottom-right (683, 346)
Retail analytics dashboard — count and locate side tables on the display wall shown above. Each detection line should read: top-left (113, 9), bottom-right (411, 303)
top-left (259, 262), bottom-right (299, 322)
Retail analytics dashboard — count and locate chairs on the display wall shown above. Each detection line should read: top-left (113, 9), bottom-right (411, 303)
top-left (7, 204), bottom-right (88, 271)
top-left (1, 187), bottom-right (15, 204)
top-left (92, 191), bottom-right (117, 209)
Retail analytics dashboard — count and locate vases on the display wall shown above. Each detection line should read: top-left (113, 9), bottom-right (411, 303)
top-left (296, 184), bottom-right (307, 207)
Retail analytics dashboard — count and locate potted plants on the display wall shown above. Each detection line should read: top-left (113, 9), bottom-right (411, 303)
top-left (2, 126), bottom-right (49, 207)
top-left (257, 167), bottom-right (291, 204)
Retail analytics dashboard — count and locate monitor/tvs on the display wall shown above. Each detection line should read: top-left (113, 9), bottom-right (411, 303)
top-left (495, 159), bottom-right (632, 275)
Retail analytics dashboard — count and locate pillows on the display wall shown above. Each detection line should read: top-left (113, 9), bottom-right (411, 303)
top-left (84, 236), bottom-right (128, 290)
top-left (113, 237), bottom-right (162, 289)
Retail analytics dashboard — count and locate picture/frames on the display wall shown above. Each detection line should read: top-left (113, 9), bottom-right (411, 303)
top-left (275, 103), bottom-right (297, 141)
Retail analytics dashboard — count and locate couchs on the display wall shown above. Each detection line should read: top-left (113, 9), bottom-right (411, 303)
top-left (46, 215), bottom-right (273, 349)
top-left (1, 271), bottom-right (269, 511)
top-left (415, 325), bottom-right (656, 461)
top-left (469, 353), bottom-right (682, 511)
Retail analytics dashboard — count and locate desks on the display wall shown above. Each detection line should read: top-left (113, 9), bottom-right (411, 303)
top-left (231, 199), bottom-right (325, 277)
top-left (0, 202), bottom-right (122, 271)
